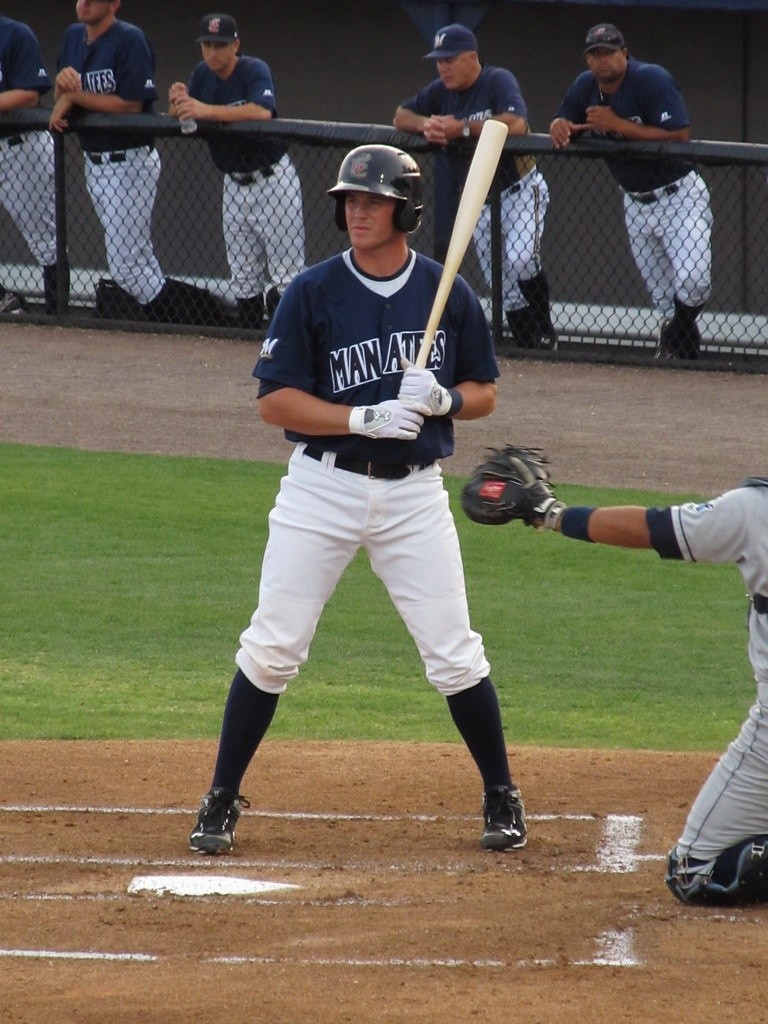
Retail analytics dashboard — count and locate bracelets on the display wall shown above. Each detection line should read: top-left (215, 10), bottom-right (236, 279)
top-left (446, 388), bottom-right (463, 417)
top-left (561, 506), bottom-right (596, 544)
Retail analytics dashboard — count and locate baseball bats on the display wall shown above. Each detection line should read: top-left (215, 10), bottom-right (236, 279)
top-left (413, 118), bottom-right (510, 372)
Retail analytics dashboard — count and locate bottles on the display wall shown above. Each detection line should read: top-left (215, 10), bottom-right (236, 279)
top-left (179, 118), bottom-right (198, 134)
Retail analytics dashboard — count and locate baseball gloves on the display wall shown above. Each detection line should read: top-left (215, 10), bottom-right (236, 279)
top-left (461, 443), bottom-right (557, 526)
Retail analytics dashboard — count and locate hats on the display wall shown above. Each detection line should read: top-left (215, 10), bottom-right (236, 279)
top-left (422, 24), bottom-right (478, 59)
top-left (195, 13), bottom-right (239, 44)
top-left (582, 23), bottom-right (625, 53)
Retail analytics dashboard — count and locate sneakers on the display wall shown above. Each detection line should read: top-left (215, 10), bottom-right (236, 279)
top-left (0, 292), bottom-right (21, 313)
top-left (481, 780), bottom-right (528, 852)
top-left (189, 786), bottom-right (250, 854)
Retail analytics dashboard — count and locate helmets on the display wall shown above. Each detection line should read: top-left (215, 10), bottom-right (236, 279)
top-left (327, 145), bottom-right (424, 233)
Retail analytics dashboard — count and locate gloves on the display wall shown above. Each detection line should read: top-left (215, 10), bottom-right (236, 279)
top-left (349, 399), bottom-right (433, 440)
top-left (395, 358), bottom-right (452, 416)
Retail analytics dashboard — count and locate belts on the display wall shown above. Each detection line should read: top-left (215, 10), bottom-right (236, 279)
top-left (484, 184), bottom-right (521, 204)
top-left (88, 144), bottom-right (155, 164)
top-left (8, 136), bottom-right (23, 146)
top-left (629, 169), bottom-right (698, 205)
top-left (229, 167), bottom-right (273, 185)
top-left (302, 444), bottom-right (435, 480)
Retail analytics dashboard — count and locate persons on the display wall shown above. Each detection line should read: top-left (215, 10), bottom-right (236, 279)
top-left (169, 13), bottom-right (305, 328)
top-left (393, 23), bottom-right (559, 350)
top-left (0, 17), bottom-right (70, 316)
top-left (551, 24), bottom-right (713, 359)
top-left (189, 144), bottom-right (528, 855)
top-left (460, 445), bottom-right (768, 912)
top-left (49, 0), bottom-right (187, 323)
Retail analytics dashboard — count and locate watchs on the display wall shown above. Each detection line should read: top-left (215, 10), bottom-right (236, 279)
top-left (462, 118), bottom-right (470, 137)
